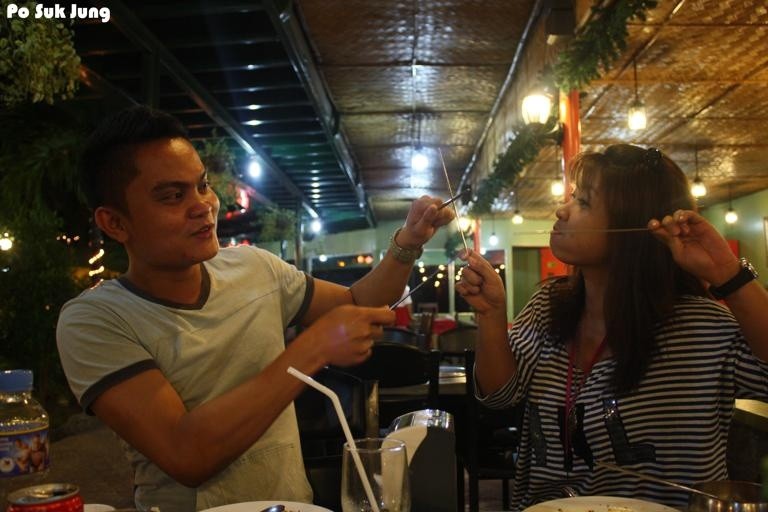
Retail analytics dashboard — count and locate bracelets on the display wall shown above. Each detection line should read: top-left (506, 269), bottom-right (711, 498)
top-left (388, 227), bottom-right (424, 264)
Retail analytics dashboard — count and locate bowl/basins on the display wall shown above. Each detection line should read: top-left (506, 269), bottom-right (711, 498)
top-left (686, 480), bottom-right (767, 512)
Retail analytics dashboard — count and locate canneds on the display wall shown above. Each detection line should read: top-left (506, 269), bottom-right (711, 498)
top-left (7, 484), bottom-right (84, 512)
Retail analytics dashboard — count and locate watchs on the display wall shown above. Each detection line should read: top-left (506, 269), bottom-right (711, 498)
top-left (709, 258), bottom-right (759, 301)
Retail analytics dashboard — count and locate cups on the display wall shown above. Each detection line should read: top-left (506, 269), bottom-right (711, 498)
top-left (340, 438), bottom-right (411, 512)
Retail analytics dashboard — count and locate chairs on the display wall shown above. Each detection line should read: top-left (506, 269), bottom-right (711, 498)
top-left (295, 349), bottom-right (525, 512)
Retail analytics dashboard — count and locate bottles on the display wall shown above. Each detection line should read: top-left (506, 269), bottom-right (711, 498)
top-left (0, 369), bottom-right (51, 493)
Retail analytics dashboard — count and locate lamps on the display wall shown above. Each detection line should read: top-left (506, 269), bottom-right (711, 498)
top-left (625, 53), bottom-right (709, 200)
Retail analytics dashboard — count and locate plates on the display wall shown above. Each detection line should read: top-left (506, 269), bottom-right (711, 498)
top-left (523, 496), bottom-right (681, 512)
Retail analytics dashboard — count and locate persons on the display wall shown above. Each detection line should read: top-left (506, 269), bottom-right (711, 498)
top-left (395, 284), bottom-right (416, 328)
top-left (56, 104), bottom-right (456, 512)
top-left (455, 144), bottom-right (768, 512)
